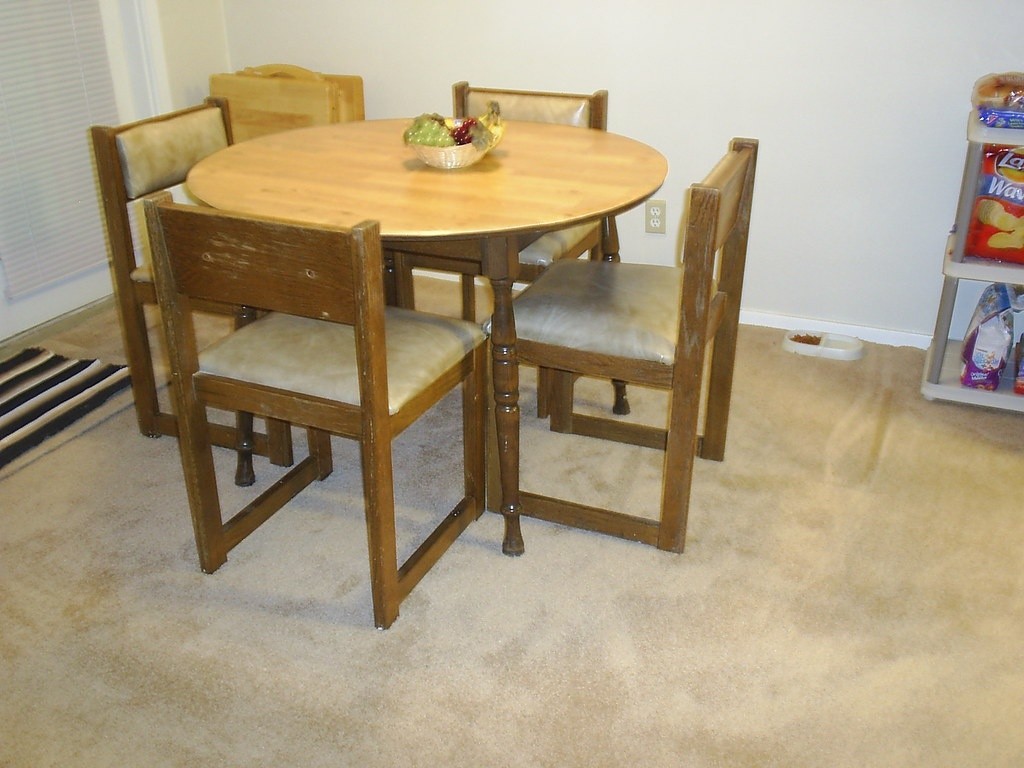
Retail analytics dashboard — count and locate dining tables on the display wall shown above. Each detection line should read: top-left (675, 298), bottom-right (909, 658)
top-left (184, 115), bottom-right (667, 557)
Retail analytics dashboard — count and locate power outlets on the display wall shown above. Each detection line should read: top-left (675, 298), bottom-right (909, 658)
top-left (644, 199), bottom-right (666, 234)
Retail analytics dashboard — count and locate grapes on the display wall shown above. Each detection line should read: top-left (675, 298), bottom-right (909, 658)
top-left (404, 114), bottom-right (475, 146)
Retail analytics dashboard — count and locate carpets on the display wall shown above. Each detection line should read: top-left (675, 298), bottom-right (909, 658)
top-left (0, 339), bottom-right (174, 482)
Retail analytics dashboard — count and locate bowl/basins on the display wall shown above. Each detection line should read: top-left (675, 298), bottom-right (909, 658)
top-left (411, 119), bottom-right (504, 170)
top-left (781, 329), bottom-right (865, 360)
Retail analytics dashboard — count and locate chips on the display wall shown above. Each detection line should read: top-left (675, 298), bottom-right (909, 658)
top-left (979, 199), bottom-right (1024, 249)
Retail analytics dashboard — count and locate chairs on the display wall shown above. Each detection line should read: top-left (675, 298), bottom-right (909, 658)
top-left (391, 80), bottom-right (633, 417)
top-left (477, 137), bottom-right (758, 556)
top-left (143, 192), bottom-right (489, 631)
top-left (90, 95), bottom-right (295, 467)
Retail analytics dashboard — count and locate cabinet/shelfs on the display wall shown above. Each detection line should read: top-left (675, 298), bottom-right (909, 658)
top-left (919, 109), bottom-right (1024, 416)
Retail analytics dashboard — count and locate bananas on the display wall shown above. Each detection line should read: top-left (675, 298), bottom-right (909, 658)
top-left (444, 100), bottom-right (503, 151)
